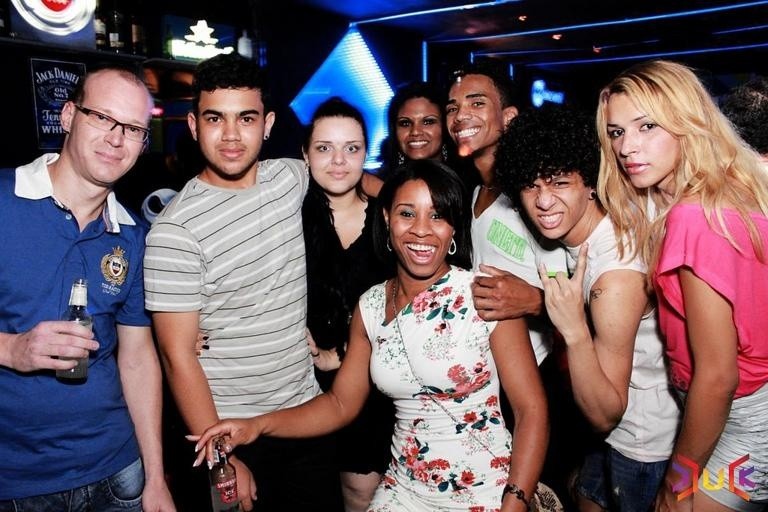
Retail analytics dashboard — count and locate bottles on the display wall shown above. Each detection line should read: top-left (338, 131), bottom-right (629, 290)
top-left (206, 437), bottom-right (238, 510)
top-left (94, 0), bottom-right (139, 55)
top-left (56, 275), bottom-right (94, 385)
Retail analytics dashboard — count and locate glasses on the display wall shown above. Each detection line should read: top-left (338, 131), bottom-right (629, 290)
top-left (75, 104), bottom-right (150, 142)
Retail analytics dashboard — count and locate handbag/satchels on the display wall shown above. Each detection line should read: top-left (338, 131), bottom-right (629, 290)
top-left (531, 482), bottom-right (562, 511)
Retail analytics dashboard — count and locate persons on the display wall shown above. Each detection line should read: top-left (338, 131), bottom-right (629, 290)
top-left (0, 53), bottom-right (765, 512)
